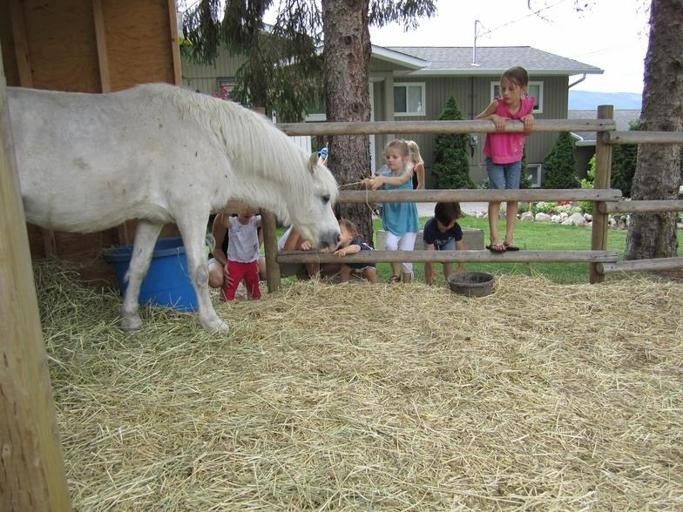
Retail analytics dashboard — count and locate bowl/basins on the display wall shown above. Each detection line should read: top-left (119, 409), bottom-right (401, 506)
top-left (446, 270), bottom-right (496, 298)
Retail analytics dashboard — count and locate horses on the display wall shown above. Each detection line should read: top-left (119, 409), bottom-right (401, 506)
top-left (4, 80), bottom-right (343, 337)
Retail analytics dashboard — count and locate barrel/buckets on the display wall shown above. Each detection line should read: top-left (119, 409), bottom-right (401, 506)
top-left (103, 236), bottom-right (197, 314)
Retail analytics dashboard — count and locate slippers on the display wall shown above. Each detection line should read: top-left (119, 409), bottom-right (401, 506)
top-left (485, 245), bottom-right (506, 253)
top-left (388, 275), bottom-right (400, 283)
top-left (506, 246), bottom-right (519, 250)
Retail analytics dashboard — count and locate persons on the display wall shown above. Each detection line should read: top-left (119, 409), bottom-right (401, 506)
top-left (405, 141), bottom-right (425, 190)
top-left (278, 224), bottom-right (323, 283)
top-left (207, 213), bottom-right (266, 288)
top-left (423, 202), bottom-right (466, 286)
top-left (333, 218), bottom-right (379, 284)
top-left (220, 212), bottom-right (262, 302)
top-left (361, 139), bottom-right (420, 284)
top-left (474, 66), bottom-right (535, 253)
